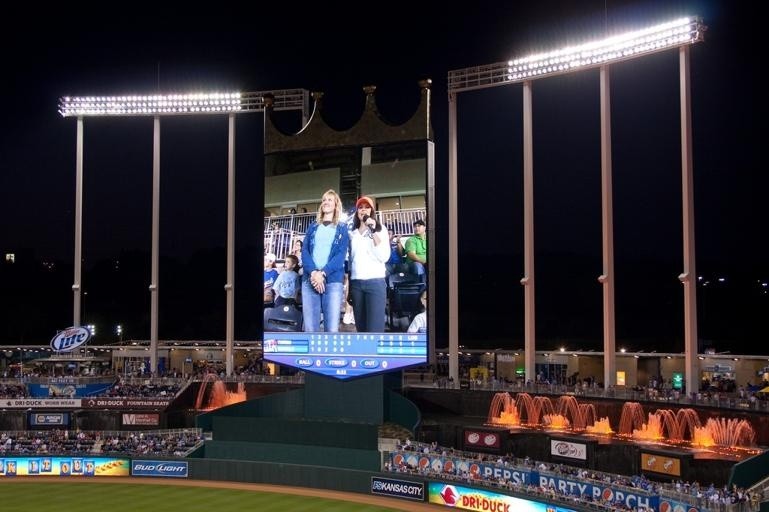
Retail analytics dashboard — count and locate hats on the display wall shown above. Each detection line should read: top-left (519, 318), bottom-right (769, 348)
top-left (413, 220), bottom-right (426, 227)
top-left (355, 196), bottom-right (374, 211)
top-left (264, 253), bottom-right (276, 263)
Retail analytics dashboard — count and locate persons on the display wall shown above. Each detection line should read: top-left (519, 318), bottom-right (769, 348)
top-left (299, 188), bottom-right (350, 333)
top-left (345, 196), bottom-right (392, 333)
top-left (265, 207), bottom-right (427, 333)
top-left (0, 357), bottom-right (272, 457)
top-left (383, 367), bottom-right (769, 512)
